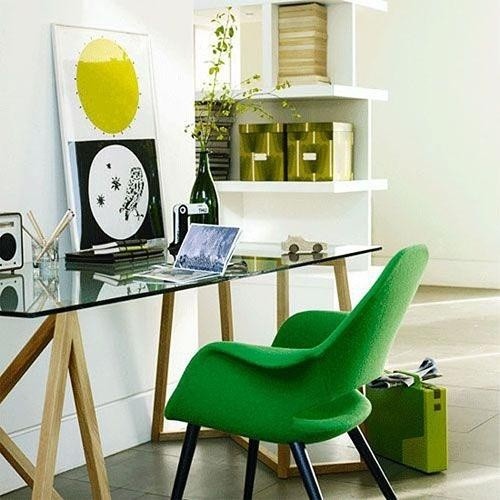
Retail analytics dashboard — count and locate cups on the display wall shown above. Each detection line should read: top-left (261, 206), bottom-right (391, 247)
top-left (32, 268), bottom-right (60, 302)
top-left (31, 236), bottom-right (59, 278)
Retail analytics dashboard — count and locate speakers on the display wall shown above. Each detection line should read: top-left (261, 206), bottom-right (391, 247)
top-left (0, 273), bottom-right (25, 313)
top-left (0, 212), bottom-right (24, 275)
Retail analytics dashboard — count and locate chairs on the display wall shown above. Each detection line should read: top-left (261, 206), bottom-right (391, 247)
top-left (168, 244), bottom-right (429, 499)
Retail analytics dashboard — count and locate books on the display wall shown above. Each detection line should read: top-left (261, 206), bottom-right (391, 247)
top-left (95, 238), bottom-right (149, 254)
top-left (195, 101), bottom-right (233, 181)
top-left (278, 3), bottom-right (330, 85)
top-left (132, 222), bottom-right (249, 285)
top-left (91, 271), bottom-right (145, 287)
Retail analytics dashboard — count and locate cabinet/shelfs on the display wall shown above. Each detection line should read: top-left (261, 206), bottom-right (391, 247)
top-left (191, 1), bottom-right (388, 275)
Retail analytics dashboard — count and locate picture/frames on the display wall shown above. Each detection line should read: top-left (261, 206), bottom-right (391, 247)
top-left (51, 22), bottom-right (169, 251)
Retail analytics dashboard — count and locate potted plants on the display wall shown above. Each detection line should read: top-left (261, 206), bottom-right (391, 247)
top-left (189, 6), bottom-right (301, 224)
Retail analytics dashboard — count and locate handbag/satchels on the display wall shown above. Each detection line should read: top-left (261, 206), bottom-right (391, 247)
top-left (366, 371), bottom-right (448, 474)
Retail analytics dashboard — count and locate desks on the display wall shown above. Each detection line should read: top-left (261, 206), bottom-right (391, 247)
top-left (0, 238), bottom-right (382, 499)
